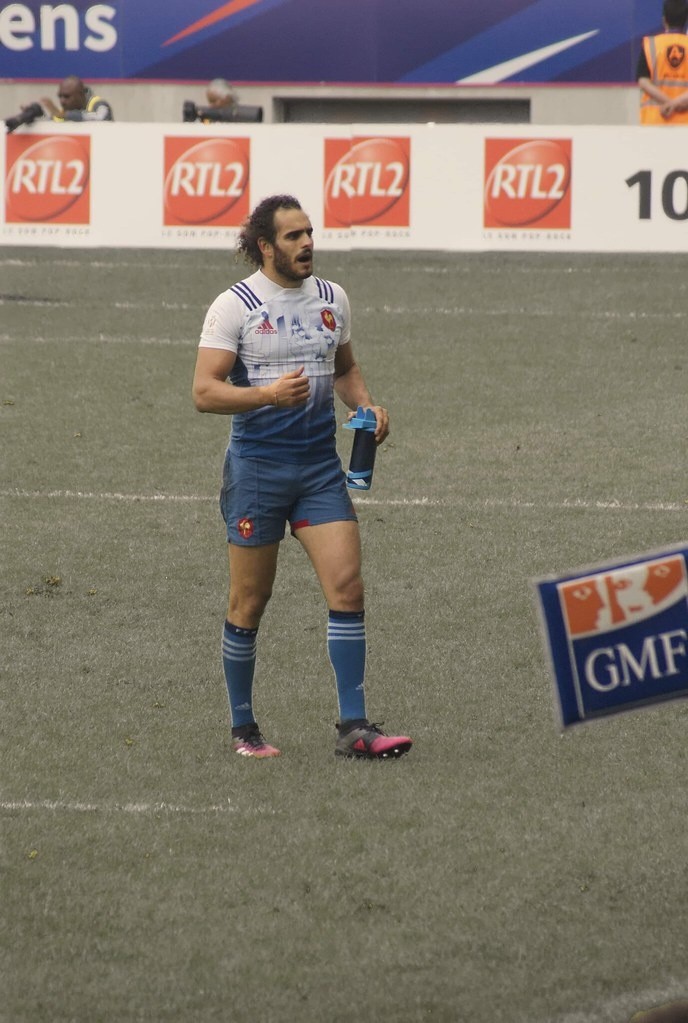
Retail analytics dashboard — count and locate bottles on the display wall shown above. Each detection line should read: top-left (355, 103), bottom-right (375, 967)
top-left (342, 406), bottom-right (377, 491)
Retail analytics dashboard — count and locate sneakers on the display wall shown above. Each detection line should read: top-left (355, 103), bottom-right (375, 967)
top-left (231, 722), bottom-right (282, 758)
top-left (335, 718), bottom-right (412, 759)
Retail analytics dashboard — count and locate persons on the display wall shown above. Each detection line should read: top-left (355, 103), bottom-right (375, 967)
top-left (636, 0), bottom-right (688, 126)
top-left (192, 195), bottom-right (412, 759)
top-left (198, 77), bottom-right (240, 124)
top-left (21, 77), bottom-right (114, 122)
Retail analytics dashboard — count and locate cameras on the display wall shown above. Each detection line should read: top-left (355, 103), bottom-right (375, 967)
top-left (4, 103), bottom-right (43, 134)
top-left (183, 101), bottom-right (263, 124)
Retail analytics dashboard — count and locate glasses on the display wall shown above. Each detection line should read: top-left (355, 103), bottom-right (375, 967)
top-left (57, 93), bottom-right (71, 99)
